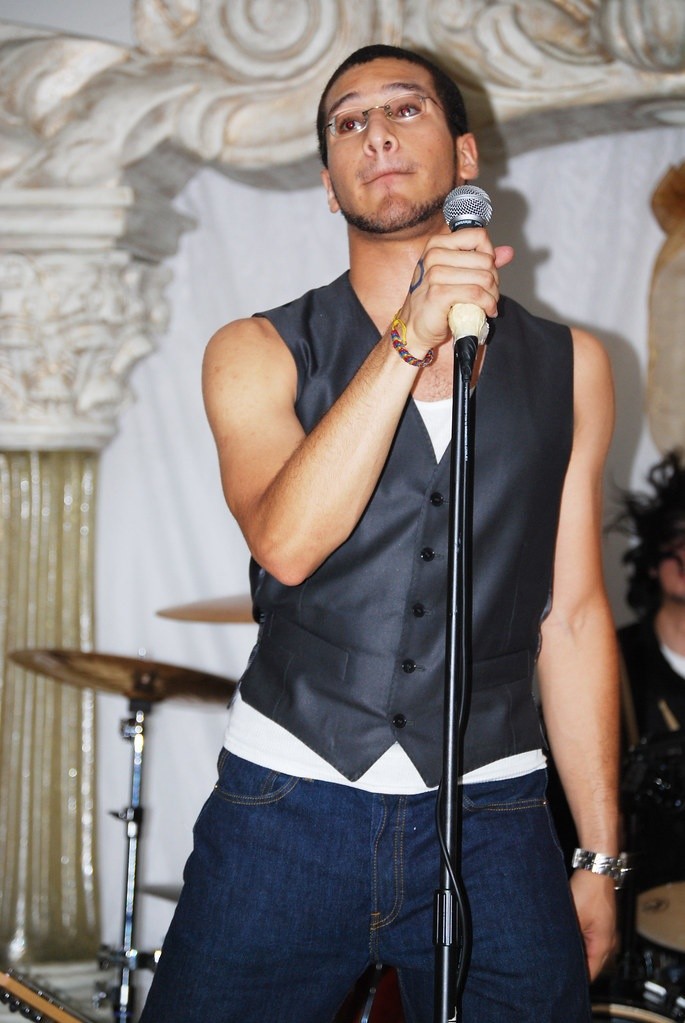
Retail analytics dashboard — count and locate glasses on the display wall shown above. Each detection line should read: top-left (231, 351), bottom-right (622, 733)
top-left (319, 93), bottom-right (451, 140)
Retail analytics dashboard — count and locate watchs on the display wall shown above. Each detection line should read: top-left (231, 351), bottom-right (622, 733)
top-left (572, 847), bottom-right (627, 890)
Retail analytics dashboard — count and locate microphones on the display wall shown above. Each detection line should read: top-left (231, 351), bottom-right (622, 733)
top-left (443, 183), bottom-right (494, 383)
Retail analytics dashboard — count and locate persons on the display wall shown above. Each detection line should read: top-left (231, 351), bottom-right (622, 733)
top-left (144, 46), bottom-right (622, 1022)
top-left (536, 446), bottom-right (684, 1004)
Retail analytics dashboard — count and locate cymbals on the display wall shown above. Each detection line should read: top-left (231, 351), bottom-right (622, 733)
top-left (7, 647), bottom-right (237, 709)
top-left (155, 595), bottom-right (258, 623)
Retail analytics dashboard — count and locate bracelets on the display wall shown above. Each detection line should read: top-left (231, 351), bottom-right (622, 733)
top-left (391, 315), bottom-right (434, 368)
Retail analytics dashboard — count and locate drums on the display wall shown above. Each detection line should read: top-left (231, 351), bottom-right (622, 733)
top-left (632, 881), bottom-right (685, 1013)
